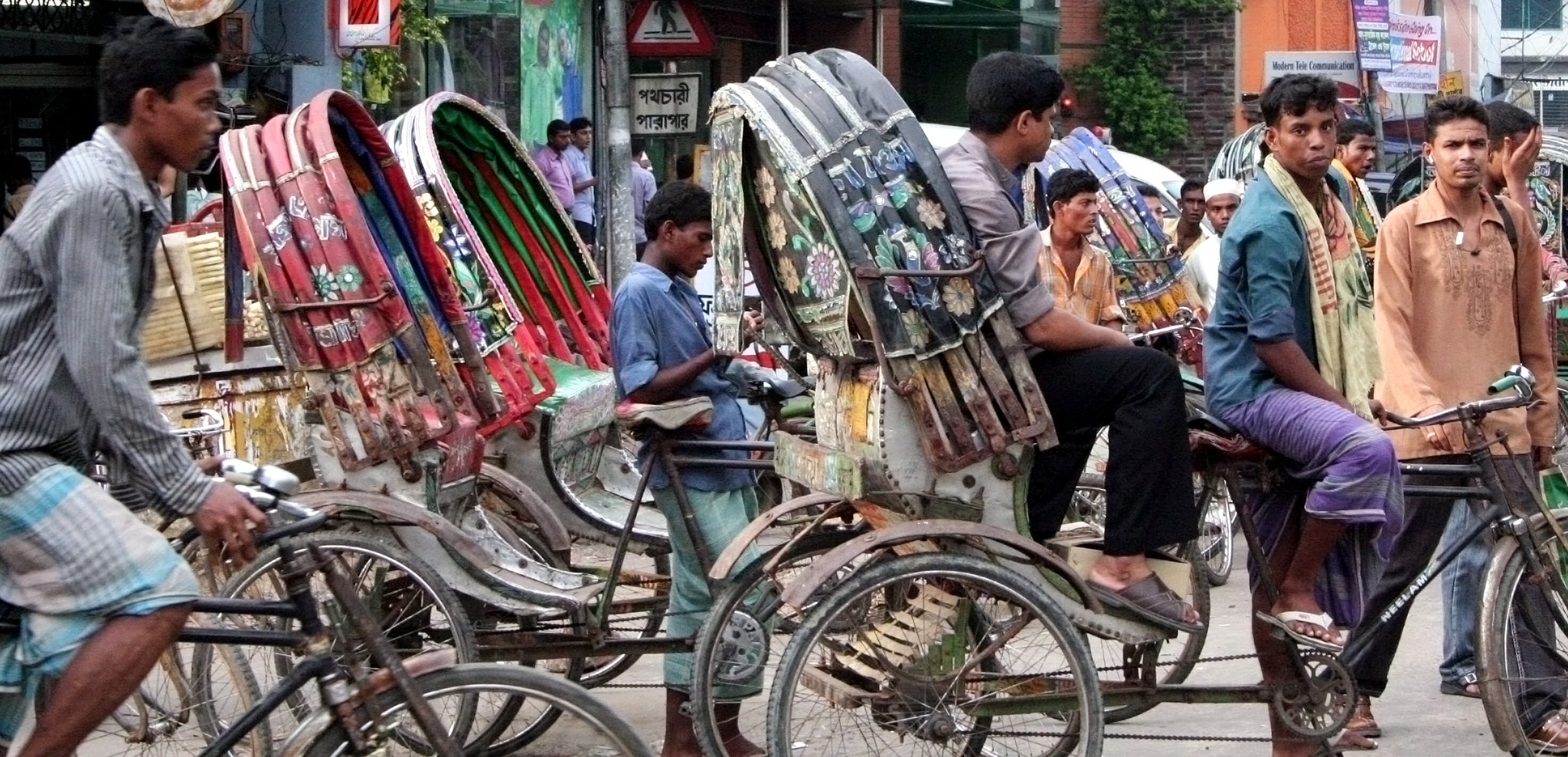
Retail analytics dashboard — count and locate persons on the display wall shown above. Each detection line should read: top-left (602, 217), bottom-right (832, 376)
top-left (1204, 74), bottom-right (1406, 757)
top-left (534, 116), bottom-right (658, 262)
top-left (938, 51), bottom-right (1210, 632)
top-left (1336, 96), bottom-right (1568, 753)
top-left (1484, 102), bottom-right (1542, 237)
top-left (0, 16), bottom-right (266, 757)
top-left (1032, 169), bottom-right (1125, 332)
top-left (1331, 118), bottom-right (1381, 263)
top-left (523, 19), bottom-right (583, 152)
top-left (0, 154), bottom-right (37, 232)
top-left (607, 180), bottom-right (772, 757)
top-left (1136, 175), bottom-right (1241, 311)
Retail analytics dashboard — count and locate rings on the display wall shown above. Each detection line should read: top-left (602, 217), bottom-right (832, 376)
top-left (1427, 432), bottom-right (1436, 438)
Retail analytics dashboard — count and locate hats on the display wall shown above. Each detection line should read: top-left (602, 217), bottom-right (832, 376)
top-left (1204, 178), bottom-right (1244, 203)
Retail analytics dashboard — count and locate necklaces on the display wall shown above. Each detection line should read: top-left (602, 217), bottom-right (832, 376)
top-left (1430, 154), bottom-right (1482, 244)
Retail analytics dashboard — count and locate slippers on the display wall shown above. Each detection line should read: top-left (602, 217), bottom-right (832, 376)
top-left (1086, 570), bottom-right (1205, 634)
top-left (1310, 742), bottom-right (1344, 757)
top-left (1256, 610), bottom-right (1344, 653)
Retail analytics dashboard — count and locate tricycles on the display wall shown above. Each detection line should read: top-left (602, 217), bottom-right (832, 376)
top-left (0, 47), bottom-right (1568, 757)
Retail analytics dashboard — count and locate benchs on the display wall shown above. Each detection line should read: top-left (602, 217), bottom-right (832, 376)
top-left (489, 355), bottom-right (615, 496)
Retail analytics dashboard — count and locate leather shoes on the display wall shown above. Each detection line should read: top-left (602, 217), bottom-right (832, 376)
top-left (1527, 712), bottom-right (1568, 753)
top-left (1332, 691), bottom-right (1381, 737)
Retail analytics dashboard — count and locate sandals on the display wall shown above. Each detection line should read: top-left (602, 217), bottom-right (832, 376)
top-left (1440, 672), bottom-right (1484, 698)
